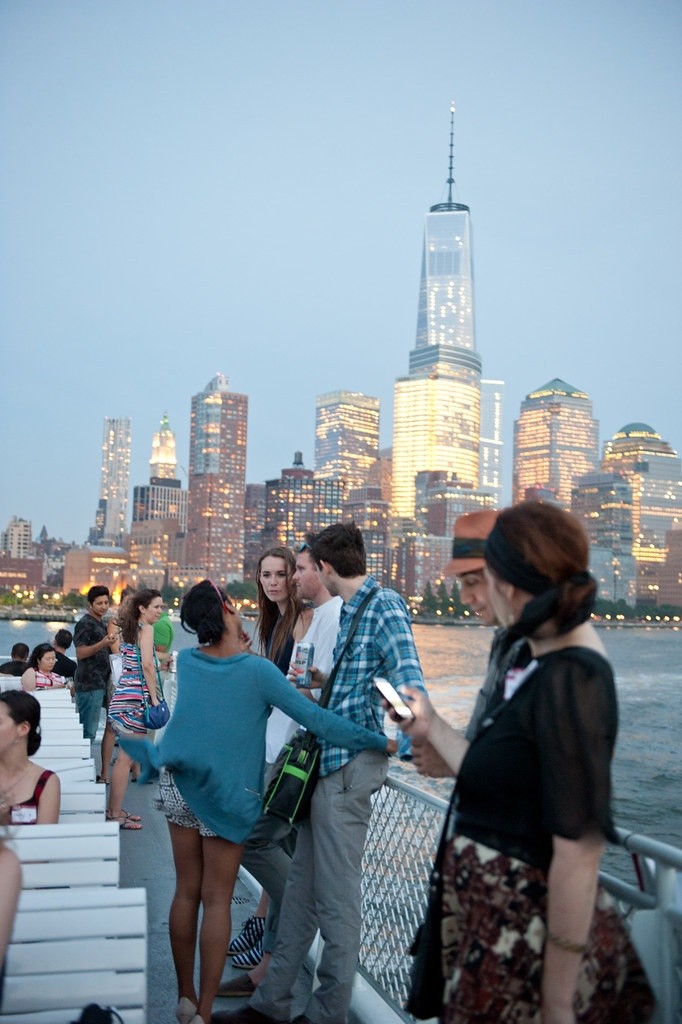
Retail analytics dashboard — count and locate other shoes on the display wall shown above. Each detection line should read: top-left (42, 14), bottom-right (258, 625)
top-left (232, 937), bottom-right (263, 968)
top-left (217, 974), bottom-right (256, 996)
top-left (177, 997), bottom-right (197, 1024)
top-left (227, 916), bottom-right (266, 956)
top-left (189, 1015), bottom-right (205, 1024)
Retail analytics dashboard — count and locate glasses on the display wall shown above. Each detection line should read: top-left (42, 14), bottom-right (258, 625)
top-left (297, 542), bottom-right (310, 553)
top-left (183, 578), bottom-right (227, 614)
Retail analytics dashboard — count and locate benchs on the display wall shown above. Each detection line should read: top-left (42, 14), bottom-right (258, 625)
top-left (0, 674), bottom-right (146, 1024)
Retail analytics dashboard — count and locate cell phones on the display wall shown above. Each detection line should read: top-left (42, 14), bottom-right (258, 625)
top-left (374, 676), bottom-right (416, 722)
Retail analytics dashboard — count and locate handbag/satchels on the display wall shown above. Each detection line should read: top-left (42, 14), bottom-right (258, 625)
top-left (405, 889), bottom-right (444, 1017)
top-left (144, 700), bottom-right (170, 729)
top-left (263, 728), bottom-right (320, 824)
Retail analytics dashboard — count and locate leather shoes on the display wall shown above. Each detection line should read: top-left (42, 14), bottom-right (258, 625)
top-left (212, 1005), bottom-right (289, 1024)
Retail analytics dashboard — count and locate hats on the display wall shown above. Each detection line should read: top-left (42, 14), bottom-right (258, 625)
top-left (443, 511), bottom-right (499, 573)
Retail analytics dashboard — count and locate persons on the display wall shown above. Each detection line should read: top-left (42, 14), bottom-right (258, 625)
top-left (216, 544), bottom-right (344, 999)
top-left (381, 503), bottom-right (662, 1024)
top-left (0, 628), bottom-right (78, 692)
top-left (73, 585), bottom-right (175, 830)
top-left (228, 546), bottom-right (313, 970)
top-left (0, 836), bottom-right (22, 1009)
top-left (0, 689), bottom-right (70, 891)
top-left (117, 581), bottom-right (398, 1024)
top-left (211, 519), bottom-right (429, 1024)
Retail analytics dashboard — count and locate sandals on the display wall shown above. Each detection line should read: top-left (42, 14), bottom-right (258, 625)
top-left (106, 809), bottom-right (142, 830)
